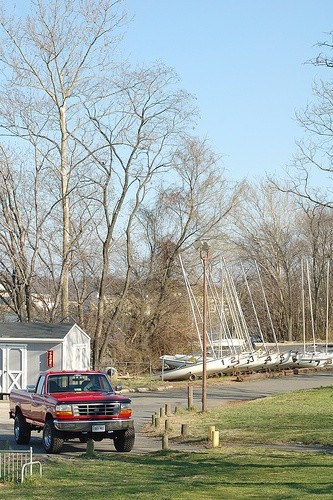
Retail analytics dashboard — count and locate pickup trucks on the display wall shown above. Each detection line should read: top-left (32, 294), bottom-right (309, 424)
top-left (9, 369), bottom-right (136, 453)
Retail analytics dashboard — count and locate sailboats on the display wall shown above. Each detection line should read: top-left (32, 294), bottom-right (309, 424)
top-left (151, 250), bottom-right (333, 382)
